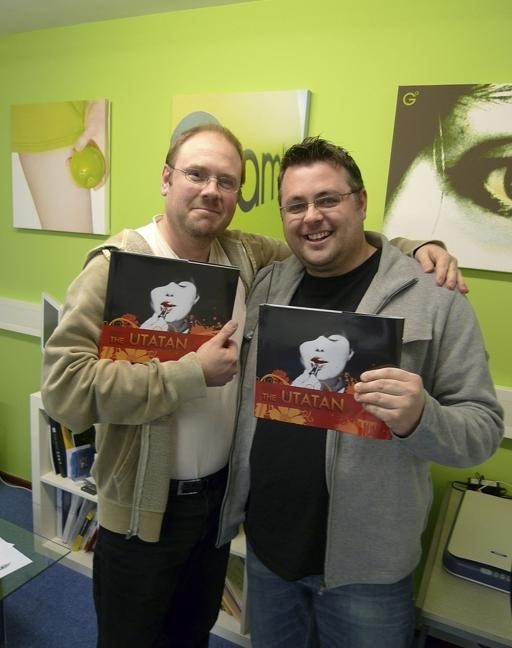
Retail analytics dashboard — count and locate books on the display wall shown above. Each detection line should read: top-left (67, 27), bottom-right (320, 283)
top-left (253, 303), bottom-right (405, 440)
top-left (221, 554), bottom-right (244, 621)
top-left (98, 252), bottom-right (239, 363)
top-left (47, 414), bottom-right (98, 554)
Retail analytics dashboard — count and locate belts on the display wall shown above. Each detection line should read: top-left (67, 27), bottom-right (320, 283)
top-left (171, 479), bottom-right (208, 496)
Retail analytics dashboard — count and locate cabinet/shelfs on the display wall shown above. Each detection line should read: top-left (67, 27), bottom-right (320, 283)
top-left (29, 392), bottom-right (251, 646)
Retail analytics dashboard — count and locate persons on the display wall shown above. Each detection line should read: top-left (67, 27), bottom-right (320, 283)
top-left (11, 99), bottom-right (108, 234)
top-left (39, 122), bottom-right (470, 648)
top-left (290, 311), bottom-right (360, 396)
top-left (138, 260), bottom-right (204, 335)
top-left (215, 136), bottom-right (506, 648)
top-left (384, 84), bottom-right (512, 272)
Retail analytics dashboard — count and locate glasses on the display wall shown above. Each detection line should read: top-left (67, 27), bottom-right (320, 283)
top-left (279, 188), bottom-right (355, 213)
top-left (168, 162), bottom-right (240, 192)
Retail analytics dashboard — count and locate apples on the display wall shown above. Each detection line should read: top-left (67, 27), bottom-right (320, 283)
top-left (70, 145), bottom-right (105, 188)
